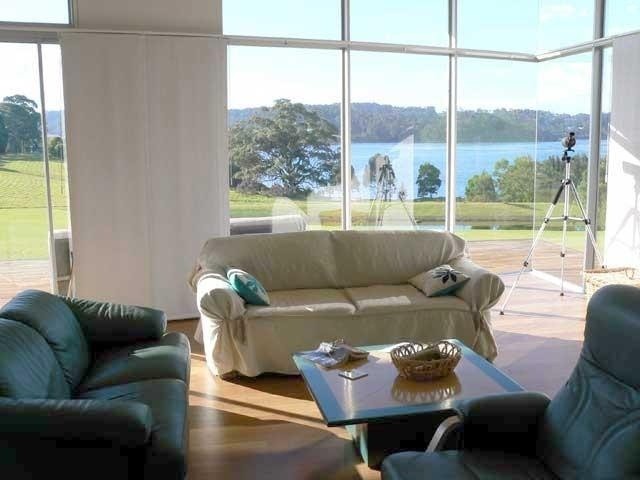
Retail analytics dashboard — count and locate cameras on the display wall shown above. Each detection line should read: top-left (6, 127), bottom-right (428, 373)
top-left (562, 132), bottom-right (575, 150)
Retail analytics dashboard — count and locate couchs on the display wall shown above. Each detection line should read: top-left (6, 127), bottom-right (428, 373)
top-left (195, 228), bottom-right (505, 380)
top-left (0, 288), bottom-right (193, 480)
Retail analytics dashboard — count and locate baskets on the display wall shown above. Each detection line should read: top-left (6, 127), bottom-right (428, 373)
top-left (390, 340), bottom-right (461, 380)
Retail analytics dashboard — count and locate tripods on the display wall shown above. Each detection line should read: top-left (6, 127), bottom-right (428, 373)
top-left (500, 150), bottom-right (607, 315)
top-left (369, 163), bottom-right (416, 228)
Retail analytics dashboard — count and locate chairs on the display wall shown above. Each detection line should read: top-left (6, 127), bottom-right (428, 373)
top-left (380, 283), bottom-right (640, 480)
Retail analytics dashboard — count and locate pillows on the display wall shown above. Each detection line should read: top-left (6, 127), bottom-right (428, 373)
top-left (408, 263), bottom-right (471, 298)
top-left (221, 264), bottom-right (272, 308)
